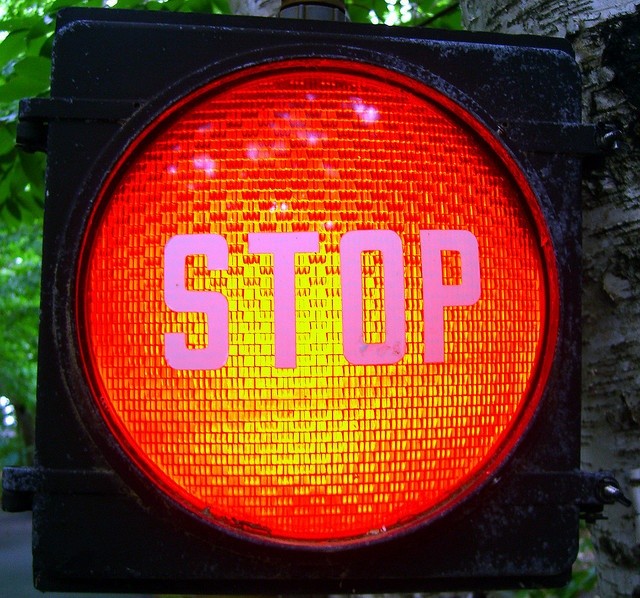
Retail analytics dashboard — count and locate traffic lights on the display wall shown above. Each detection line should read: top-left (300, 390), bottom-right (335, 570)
top-left (46, 9), bottom-right (582, 593)
